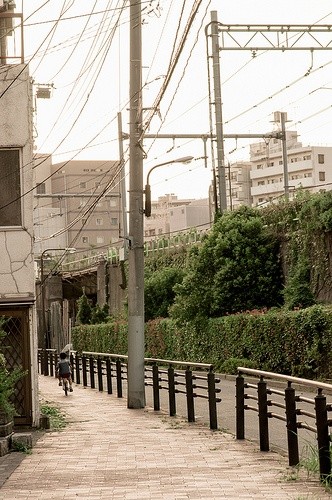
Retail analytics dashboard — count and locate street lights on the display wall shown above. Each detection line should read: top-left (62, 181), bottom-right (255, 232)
top-left (126, 154), bottom-right (195, 408)
top-left (39, 248), bottom-right (76, 375)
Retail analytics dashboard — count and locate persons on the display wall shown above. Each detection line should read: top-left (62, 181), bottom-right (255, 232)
top-left (55, 353), bottom-right (73, 392)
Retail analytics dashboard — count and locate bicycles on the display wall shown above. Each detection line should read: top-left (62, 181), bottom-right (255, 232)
top-left (56, 367), bottom-right (72, 396)
